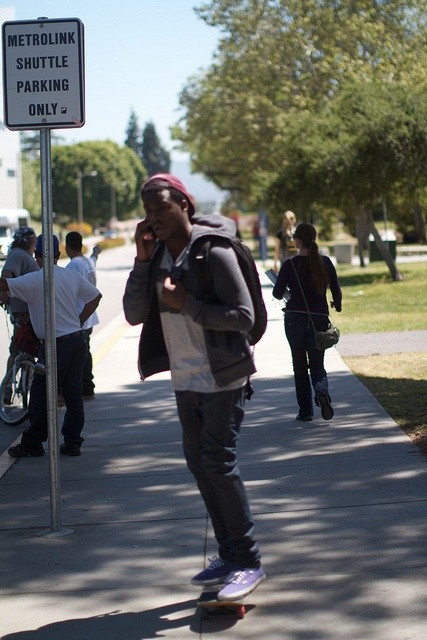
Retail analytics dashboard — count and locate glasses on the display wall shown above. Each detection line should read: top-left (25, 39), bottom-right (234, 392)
top-left (292, 233), bottom-right (296, 237)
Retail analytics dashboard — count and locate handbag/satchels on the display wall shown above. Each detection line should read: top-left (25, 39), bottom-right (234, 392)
top-left (289, 257), bottom-right (341, 352)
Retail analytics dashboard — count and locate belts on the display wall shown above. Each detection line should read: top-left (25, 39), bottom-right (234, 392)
top-left (38, 330), bottom-right (89, 343)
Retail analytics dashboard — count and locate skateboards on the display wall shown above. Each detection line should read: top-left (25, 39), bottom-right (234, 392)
top-left (196, 584), bottom-right (246, 620)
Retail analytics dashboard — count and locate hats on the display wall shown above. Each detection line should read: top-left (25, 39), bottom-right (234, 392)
top-left (36, 235), bottom-right (60, 257)
top-left (295, 223), bottom-right (317, 241)
top-left (141, 173), bottom-right (196, 218)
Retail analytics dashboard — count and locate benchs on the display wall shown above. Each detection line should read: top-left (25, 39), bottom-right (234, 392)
top-left (319, 239), bottom-right (358, 263)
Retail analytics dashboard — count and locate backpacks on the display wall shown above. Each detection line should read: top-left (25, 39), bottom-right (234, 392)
top-left (194, 236), bottom-right (269, 347)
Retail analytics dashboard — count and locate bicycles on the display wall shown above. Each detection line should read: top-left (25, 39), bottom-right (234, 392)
top-left (0, 301), bottom-right (36, 426)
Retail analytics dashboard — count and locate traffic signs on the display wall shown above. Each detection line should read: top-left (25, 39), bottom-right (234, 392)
top-left (2, 18), bottom-right (82, 130)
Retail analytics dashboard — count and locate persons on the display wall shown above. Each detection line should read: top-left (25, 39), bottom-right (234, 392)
top-left (273, 225), bottom-right (342, 422)
top-left (123, 172), bottom-right (267, 602)
top-left (0, 234), bottom-right (102, 459)
top-left (0, 227), bottom-right (39, 404)
top-left (58, 232), bottom-right (101, 405)
top-left (274, 211), bottom-right (302, 276)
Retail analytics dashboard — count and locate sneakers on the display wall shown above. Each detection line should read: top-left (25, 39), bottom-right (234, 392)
top-left (59, 444), bottom-right (82, 457)
top-left (191, 556), bottom-right (235, 586)
top-left (296, 414), bottom-right (314, 421)
top-left (215, 566), bottom-right (266, 601)
top-left (8, 444), bottom-right (47, 458)
top-left (317, 391), bottom-right (335, 420)
